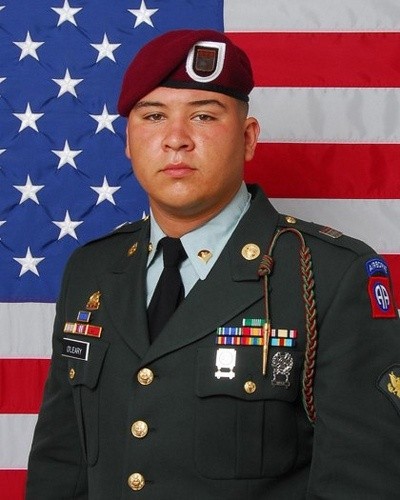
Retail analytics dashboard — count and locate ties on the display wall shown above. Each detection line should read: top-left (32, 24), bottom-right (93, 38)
top-left (146, 236), bottom-right (190, 346)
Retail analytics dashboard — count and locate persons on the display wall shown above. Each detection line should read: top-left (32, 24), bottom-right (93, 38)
top-left (21, 29), bottom-right (400, 500)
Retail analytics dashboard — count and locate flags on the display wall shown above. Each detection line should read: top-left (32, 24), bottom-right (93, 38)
top-left (0, 0), bottom-right (400, 500)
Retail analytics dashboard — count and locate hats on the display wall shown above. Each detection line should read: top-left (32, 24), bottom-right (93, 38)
top-left (117, 29), bottom-right (255, 117)
